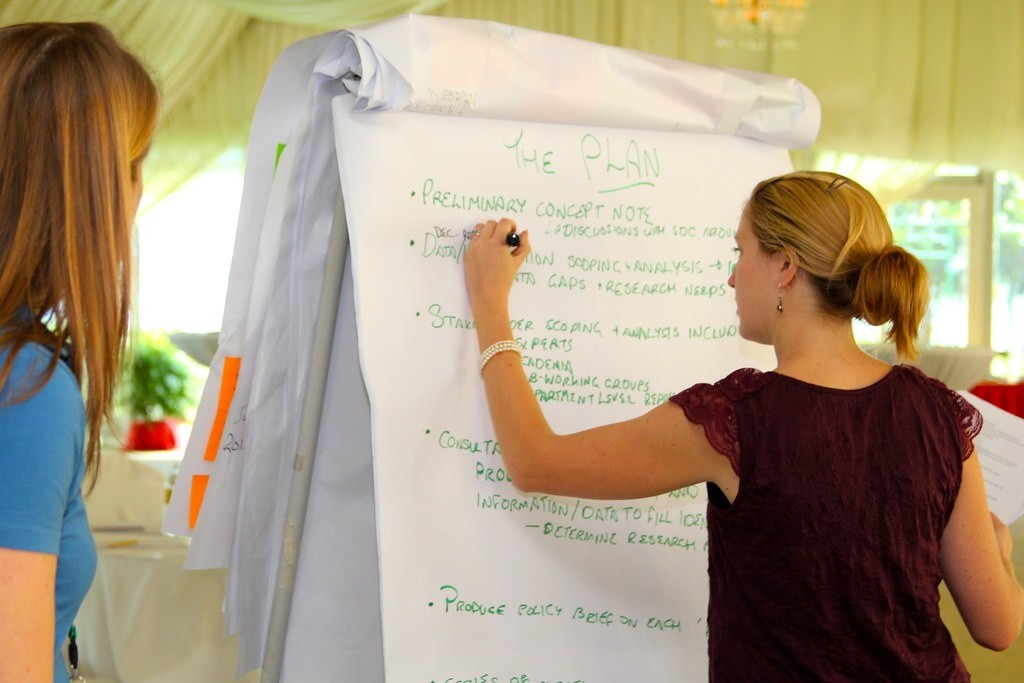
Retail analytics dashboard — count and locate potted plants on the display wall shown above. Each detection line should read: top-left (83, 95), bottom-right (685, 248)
top-left (120, 340), bottom-right (194, 450)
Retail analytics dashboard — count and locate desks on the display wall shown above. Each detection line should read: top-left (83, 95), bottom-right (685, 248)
top-left (74, 523), bottom-right (263, 682)
top-left (79, 418), bottom-right (193, 527)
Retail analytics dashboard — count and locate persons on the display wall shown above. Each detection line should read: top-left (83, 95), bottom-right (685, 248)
top-left (1, 20), bottom-right (158, 682)
top-left (463, 172), bottom-right (1024, 683)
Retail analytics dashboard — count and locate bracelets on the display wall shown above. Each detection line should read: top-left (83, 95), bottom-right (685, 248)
top-left (479, 340), bottom-right (523, 378)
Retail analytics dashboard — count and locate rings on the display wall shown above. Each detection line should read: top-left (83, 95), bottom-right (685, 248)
top-left (470, 230), bottom-right (481, 239)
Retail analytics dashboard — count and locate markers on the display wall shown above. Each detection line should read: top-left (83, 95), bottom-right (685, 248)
top-left (506, 234), bottom-right (520, 247)
